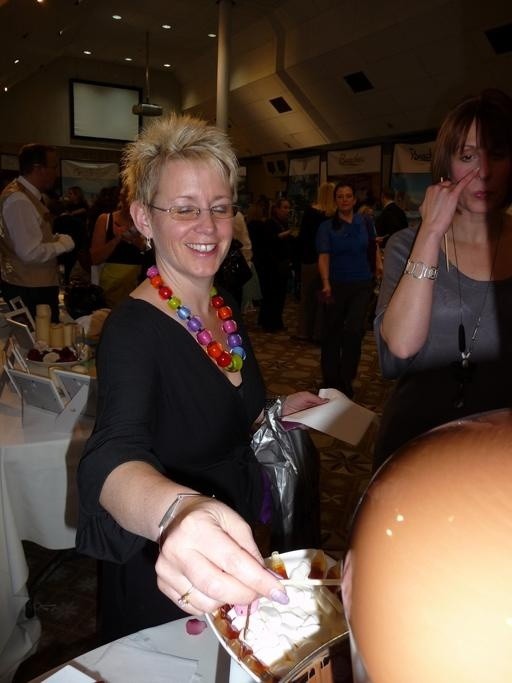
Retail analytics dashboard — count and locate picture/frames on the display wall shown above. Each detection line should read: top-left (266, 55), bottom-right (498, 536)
top-left (0, 293), bottom-right (99, 429)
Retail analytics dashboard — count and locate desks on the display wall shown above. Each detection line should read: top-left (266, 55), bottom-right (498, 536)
top-left (0, 349), bottom-right (103, 653)
top-left (21, 607), bottom-right (234, 682)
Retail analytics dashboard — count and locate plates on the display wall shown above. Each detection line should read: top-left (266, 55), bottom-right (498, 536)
top-left (27, 347), bottom-right (89, 366)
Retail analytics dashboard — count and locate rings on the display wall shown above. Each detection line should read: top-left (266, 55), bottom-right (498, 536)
top-left (178, 586), bottom-right (195, 606)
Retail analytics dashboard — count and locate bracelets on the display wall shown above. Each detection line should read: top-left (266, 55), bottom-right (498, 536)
top-left (155, 489), bottom-right (203, 553)
top-left (404, 259), bottom-right (440, 281)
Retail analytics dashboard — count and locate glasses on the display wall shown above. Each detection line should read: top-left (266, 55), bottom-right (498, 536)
top-left (143, 202), bottom-right (240, 220)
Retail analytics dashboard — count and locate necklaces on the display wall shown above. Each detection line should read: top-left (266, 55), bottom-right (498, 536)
top-left (449, 209), bottom-right (506, 369)
top-left (145, 265), bottom-right (246, 371)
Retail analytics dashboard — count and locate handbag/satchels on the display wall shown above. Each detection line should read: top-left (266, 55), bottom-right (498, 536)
top-left (64, 279), bottom-right (105, 319)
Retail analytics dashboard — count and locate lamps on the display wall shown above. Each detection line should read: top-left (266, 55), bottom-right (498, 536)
top-left (131, 25), bottom-right (164, 119)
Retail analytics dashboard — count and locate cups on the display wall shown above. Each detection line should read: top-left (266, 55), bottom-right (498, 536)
top-left (34, 303), bottom-right (84, 349)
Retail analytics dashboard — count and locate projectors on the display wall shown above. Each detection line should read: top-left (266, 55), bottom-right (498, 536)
top-left (133, 104), bottom-right (162, 116)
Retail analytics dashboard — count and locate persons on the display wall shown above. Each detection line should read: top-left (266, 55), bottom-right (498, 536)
top-left (340, 404), bottom-right (512, 683)
top-left (75, 109), bottom-right (330, 650)
top-left (2, 141), bottom-right (156, 325)
top-left (215, 182), bottom-right (409, 402)
top-left (373, 98), bottom-right (512, 476)
